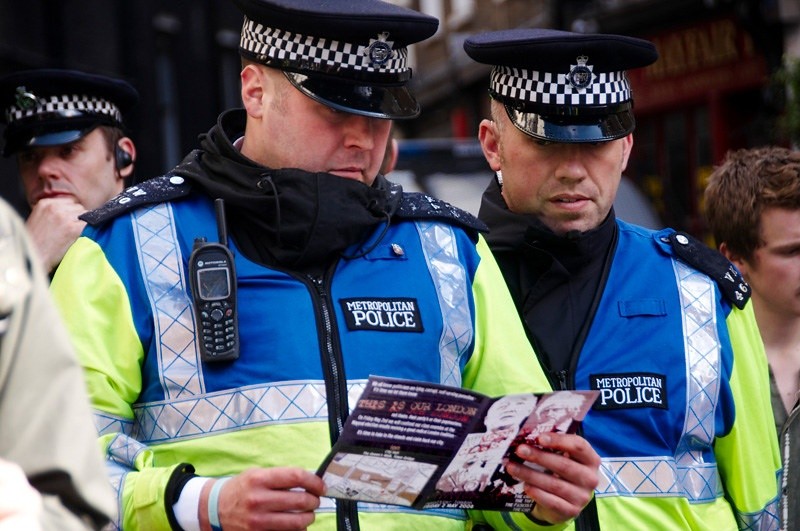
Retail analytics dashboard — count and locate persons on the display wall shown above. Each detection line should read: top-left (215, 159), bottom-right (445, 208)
top-left (7, 65), bottom-right (136, 295)
top-left (47, 0), bottom-right (601, 531)
top-left (435, 391), bottom-right (586, 494)
top-left (701, 144), bottom-right (800, 531)
top-left (0, 198), bottom-right (120, 531)
top-left (465, 28), bottom-right (781, 531)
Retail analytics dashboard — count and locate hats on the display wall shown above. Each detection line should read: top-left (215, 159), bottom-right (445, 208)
top-left (463, 28), bottom-right (662, 142)
top-left (238, 1), bottom-right (439, 119)
top-left (0, 68), bottom-right (150, 151)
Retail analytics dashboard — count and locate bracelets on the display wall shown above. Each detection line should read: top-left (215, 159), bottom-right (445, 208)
top-left (208, 476), bottom-right (233, 531)
